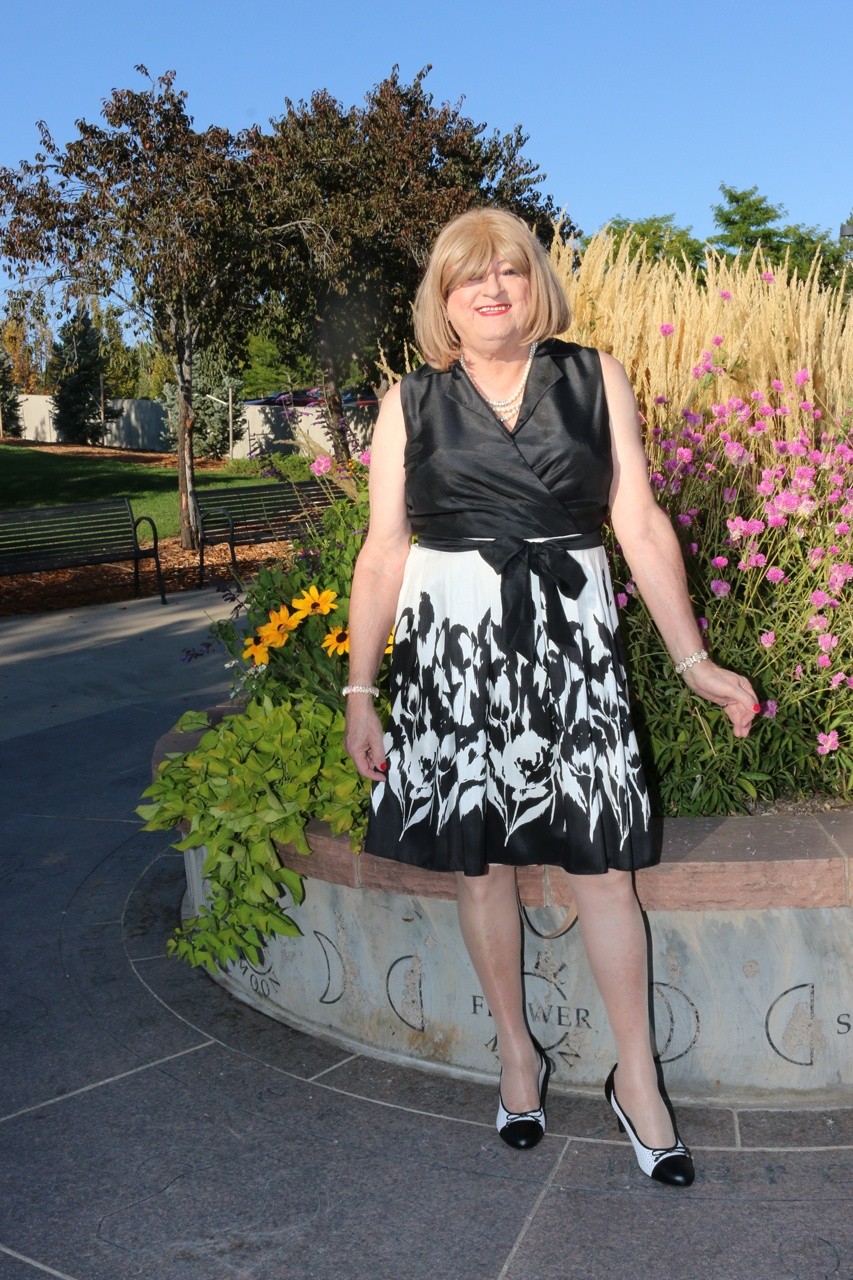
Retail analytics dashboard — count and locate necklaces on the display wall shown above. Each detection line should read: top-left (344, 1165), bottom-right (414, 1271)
top-left (453, 339), bottom-right (540, 426)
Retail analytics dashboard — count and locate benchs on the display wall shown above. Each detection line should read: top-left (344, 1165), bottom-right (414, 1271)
top-left (182, 476), bottom-right (349, 598)
top-left (0, 498), bottom-right (170, 619)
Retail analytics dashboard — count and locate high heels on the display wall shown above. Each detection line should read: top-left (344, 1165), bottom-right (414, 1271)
top-left (495, 1035), bottom-right (551, 1151)
top-left (604, 1057), bottom-right (696, 1187)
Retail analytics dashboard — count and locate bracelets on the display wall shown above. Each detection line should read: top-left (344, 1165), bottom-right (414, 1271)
top-left (672, 647), bottom-right (710, 676)
top-left (340, 685), bottom-right (381, 699)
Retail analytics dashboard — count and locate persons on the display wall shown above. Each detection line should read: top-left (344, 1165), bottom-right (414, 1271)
top-left (336, 208), bottom-right (767, 1188)
